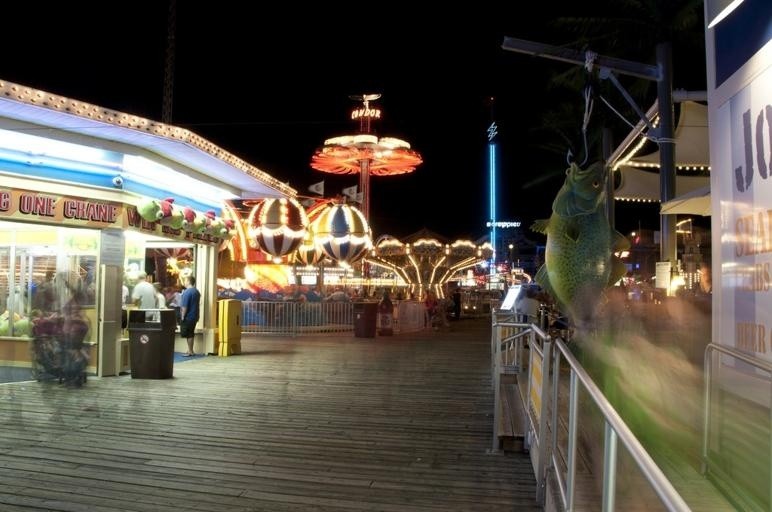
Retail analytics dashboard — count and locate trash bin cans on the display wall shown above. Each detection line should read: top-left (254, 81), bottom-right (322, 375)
top-left (128, 309), bottom-right (176, 379)
top-left (351, 302), bottom-right (378, 338)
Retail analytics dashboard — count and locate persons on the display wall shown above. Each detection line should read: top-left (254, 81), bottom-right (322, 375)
top-left (518, 287), bottom-right (540, 350)
top-left (215, 284), bottom-right (461, 332)
top-left (37, 263), bottom-right (184, 325)
top-left (176, 275), bottom-right (200, 357)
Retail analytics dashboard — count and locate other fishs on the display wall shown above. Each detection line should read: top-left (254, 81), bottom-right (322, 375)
top-left (529, 159), bottom-right (630, 368)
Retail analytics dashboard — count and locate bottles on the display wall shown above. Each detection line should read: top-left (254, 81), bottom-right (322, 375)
top-left (379, 292), bottom-right (395, 337)
